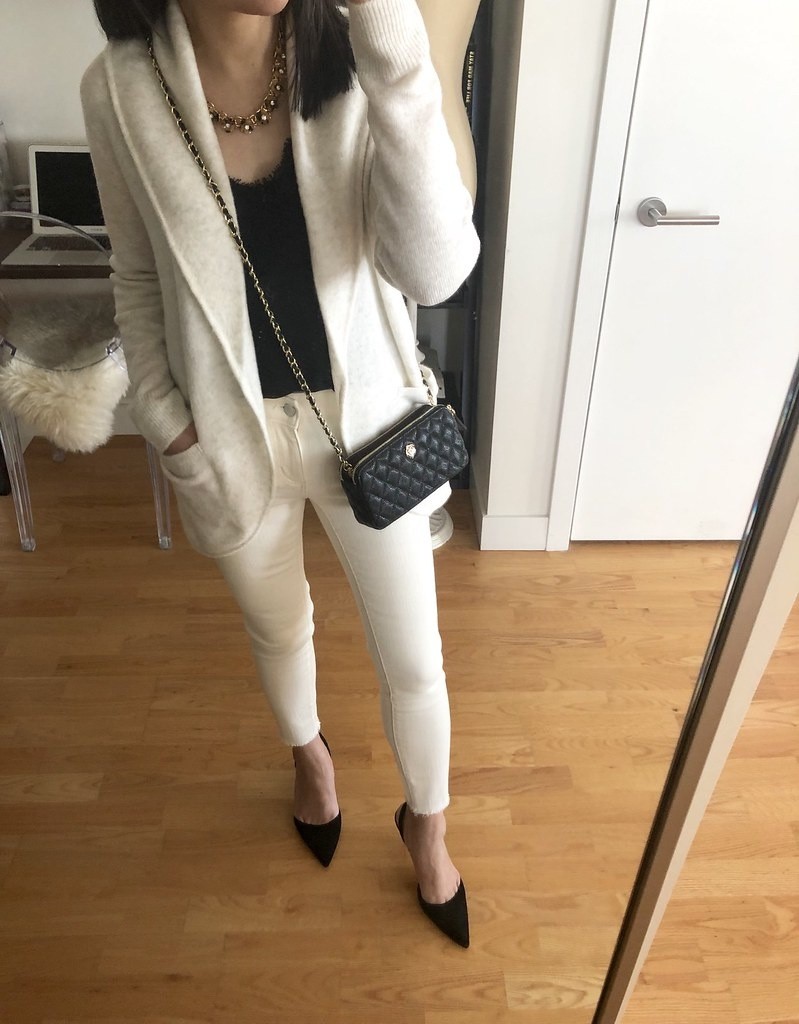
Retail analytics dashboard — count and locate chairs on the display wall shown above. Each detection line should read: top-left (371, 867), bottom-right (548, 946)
top-left (0, 210), bottom-right (174, 552)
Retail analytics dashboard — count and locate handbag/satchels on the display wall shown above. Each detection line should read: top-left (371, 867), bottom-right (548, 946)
top-left (339, 404), bottom-right (469, 530)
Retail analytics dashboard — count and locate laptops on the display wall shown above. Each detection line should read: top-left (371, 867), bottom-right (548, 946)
top-left (1, 144), bottom-right (114, 267)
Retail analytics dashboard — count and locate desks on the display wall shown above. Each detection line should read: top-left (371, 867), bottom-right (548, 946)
top-left (0, 233), bottom-right (113, 279)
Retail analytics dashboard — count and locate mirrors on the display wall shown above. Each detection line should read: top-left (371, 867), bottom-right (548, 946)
top-left (0, 1), bottom-right (798, 1024)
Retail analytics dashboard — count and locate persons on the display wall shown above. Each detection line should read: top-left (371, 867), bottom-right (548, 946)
top-left (79, 0), bottom-right (483, 946)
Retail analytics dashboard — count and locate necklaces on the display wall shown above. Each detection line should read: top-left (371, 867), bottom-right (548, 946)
top-left (197, 12), bottom-right (286, 135)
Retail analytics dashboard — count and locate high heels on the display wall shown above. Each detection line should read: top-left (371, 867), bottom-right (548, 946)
top-left (292, 732), bottom-right (342, 868)
top-left (398, 802), bottom-right (469, 948)
